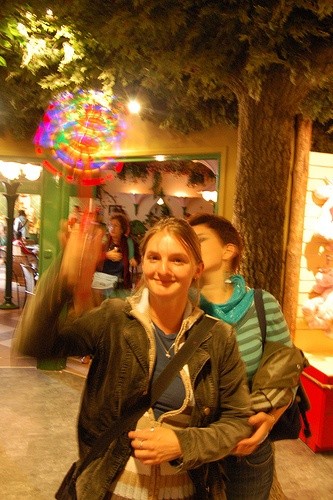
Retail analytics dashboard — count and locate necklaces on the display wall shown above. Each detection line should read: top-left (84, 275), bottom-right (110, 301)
top-left (147, 310), bottom-right (185, 359)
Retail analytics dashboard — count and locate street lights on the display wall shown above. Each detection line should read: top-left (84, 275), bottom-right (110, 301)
top-left (0, 161), bottom-right (40, 310)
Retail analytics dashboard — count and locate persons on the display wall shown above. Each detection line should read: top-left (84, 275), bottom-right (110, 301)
top-left (12, 232), bottom-right (37, 275)
top-left (62, 206), bottom-right (142, 365)
top-left (186, 214), bottom-right (300, 500)
top-left (10, 207), bottom-right (255, 500)
top-left (13, 210), bottom-right (38, 240)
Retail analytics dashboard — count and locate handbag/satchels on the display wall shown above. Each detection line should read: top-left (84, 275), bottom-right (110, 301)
top-left (268, 379), bottom-right (312, 441)
top-left (55, 461), bottom-right (77, 500)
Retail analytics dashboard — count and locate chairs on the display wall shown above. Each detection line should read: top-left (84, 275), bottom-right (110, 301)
top-left (12, 254), bottom-right (39, 310)
